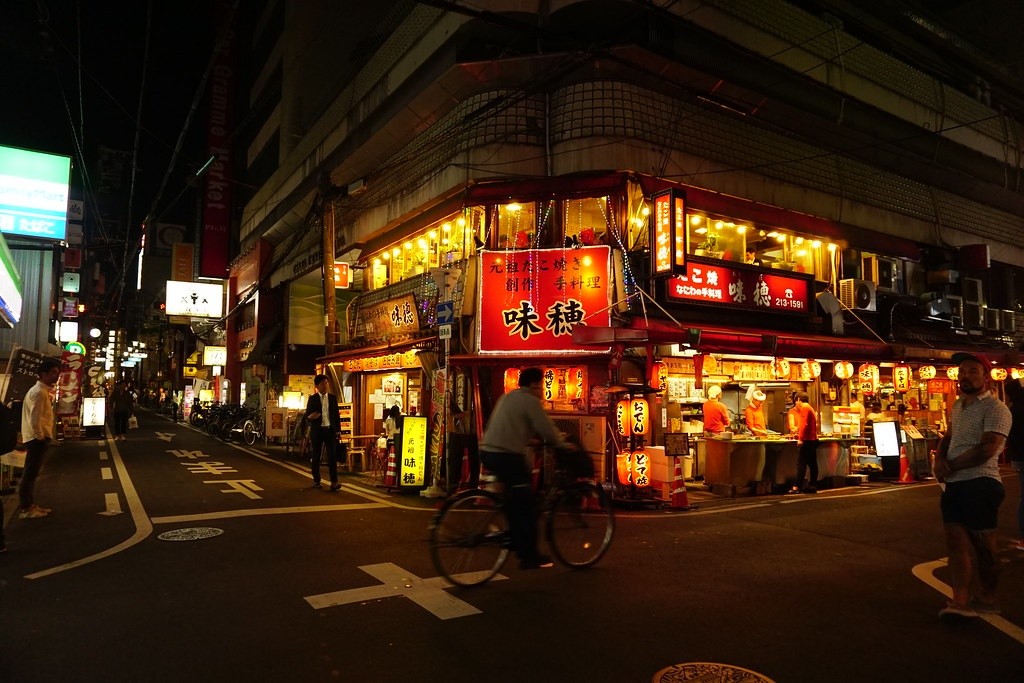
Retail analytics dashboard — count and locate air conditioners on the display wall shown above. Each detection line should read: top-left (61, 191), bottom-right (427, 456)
top-left (923, 279), bottom-right (1015, 333)
top-left (544, 414), bottom-right (606, 511)
top-left (861, 255), bottom-right (894, 293)
top-left (840, 277), bottom-right (876, 312)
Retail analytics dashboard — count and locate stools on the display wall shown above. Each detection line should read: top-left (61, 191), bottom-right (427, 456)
top-left (346, 446), bottom-right (370, 472)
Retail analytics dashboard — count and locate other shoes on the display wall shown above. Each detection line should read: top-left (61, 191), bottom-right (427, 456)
top-left (805, 487), bottom-right (818, 494)
top-left (19, 508), bottom-right (47, 519)
top-left (786, 488), bottom-right (801, 494)
top-left (521, 553), bottom-right (550, 570)
top-left (314, 481), bottom-right (322, 488)
top-left (35, 505), bottom-right (51, 513)
top-left (115, 436), bottom-right (120, 441)
top-left (970, 597), bottom-right (1001, 613)
top-left (330, 483), bottom-right (341, 490)
top-left (938, 602), bottom-right (962, 621)
top-left (122, 434), bottom-right (126, 440)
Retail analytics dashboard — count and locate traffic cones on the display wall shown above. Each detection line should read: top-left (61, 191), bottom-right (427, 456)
top-left (669, 458), bottom-right (691, 508)
top-left (582, 465), bottom-right (602, 510)
top-left (898, 446), bottom-right (917, 483)
top-left (474, 463), bottom-right (495, 506)
top-left (384, 446), bottom-right (398, 485)
top-left (447, 448), bottom-right (473, 504)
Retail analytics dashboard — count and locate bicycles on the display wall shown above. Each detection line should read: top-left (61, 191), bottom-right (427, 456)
top-left (422, 442), bottom-right (616, 588)
top-left (189, 401), bottom-right (266, 446)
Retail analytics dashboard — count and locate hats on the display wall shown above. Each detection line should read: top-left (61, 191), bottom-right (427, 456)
top-left (952, 351), bottom-right (992, 374)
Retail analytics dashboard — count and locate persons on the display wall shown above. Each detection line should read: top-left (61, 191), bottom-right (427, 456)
top-left (385, 405), bottom-right (401, 439)
top-left (788, 392), bottom-right (819, 494)
top-left (109, 380), bottom-right (133, 441)
top-left (172, 389), bottom-right (180, 422)
top-left (306, 374), bottom-right (342, 490)
top-left (867, 402), bottom-right (885, 420)
top-left (19, 362), bottom-right (60, 518)
top-left (479, 367), bottom-right (576, 570)
top-left (786, 404), bottom-right (802, 431)
top-left (933, 352), bottom-right (1013, 625)
top-left (851, 392), bottom-right (865, 418)
top-left (746, 391), bottom-right (766, 435)
top-left (703, 385), bottom-right (730, 434)
top-left (887, 394), bottom-right (895, 410)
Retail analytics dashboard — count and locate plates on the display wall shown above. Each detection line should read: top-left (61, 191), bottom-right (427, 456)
top-left (721, 432), bottom-right (732, 440)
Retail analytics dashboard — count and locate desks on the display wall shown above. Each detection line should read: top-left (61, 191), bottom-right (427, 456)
top-left (340, 434), bottom-right (387, 473)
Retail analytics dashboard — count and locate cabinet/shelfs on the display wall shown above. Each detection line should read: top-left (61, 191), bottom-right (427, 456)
top-left (339, 402), bottom-right (354, 467)
top-left (655, 382), bottom-right (722, 481)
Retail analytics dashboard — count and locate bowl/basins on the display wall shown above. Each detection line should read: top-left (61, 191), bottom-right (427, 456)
top-left (682, 409), bottom-right (702, 415)
top-left (901, 418), bottom-right (911, 425)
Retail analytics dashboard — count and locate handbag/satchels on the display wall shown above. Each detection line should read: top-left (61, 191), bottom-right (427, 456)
top-left (336, 435), bottom-right (347, 462)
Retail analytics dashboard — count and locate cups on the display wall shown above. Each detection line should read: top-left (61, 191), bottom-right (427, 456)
top-left (911, 417), bottom-right (915, 426)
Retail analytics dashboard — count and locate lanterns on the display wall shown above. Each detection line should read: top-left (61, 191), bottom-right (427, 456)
top-left (919, 365), bottom-right (936, 382)
top-left (771, 358), bottom-right (790, 377)
top-left (650, 359), bottom-right (668, 403)
top-left (990, 367), bottom-right (1007, 381)
top-left (1011, 367), bottom-right (1024, 380)
top-left (802, 361), bottom-right (821, 378)
top-left (835, 361), bottom-right (854, 380)
top-left (616, 447), bottom-right (650, 488)
top-left (946, 366), bottom-right (959, 382)
top-left (892, 363), bottom-right (912, 394)
top-left (859, 363), bottom-right (879, 396)
top-left (616, 397), bottom-right (649, 437)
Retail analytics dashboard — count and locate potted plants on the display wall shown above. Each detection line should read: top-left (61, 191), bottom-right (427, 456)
top-left (771, 256), bottom-right (798, 271)
top-left (695, 232), bottom-right (724, 259)
